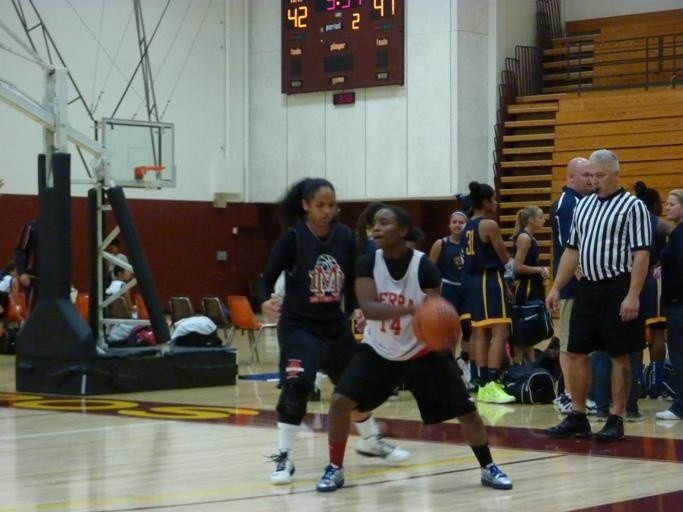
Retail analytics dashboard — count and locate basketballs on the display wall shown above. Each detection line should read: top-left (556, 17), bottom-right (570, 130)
top-left (413, 299), bottom-right (460, 350)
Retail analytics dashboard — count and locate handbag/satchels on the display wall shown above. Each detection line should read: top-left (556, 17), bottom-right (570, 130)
top-left (508, 298), bottom-right (555, 348)
top-left (497, 364), bottom-right (557, 405)
top-left (4, 277), bottom-right (29, 323)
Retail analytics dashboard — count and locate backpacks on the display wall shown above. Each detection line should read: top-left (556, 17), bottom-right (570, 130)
top-left (642, 358), bottom-right (678, 400)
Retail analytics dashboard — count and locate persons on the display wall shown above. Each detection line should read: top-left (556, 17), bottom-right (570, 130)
top-left (104, 265), bottom-right (139, 319)
top-left (106, 239), bottom-right (129, 287)
top-left (0, 264), bottom-right (19, 324)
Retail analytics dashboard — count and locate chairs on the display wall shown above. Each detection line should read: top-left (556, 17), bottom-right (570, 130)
top-left (0, 278), bottom-right (278, 369)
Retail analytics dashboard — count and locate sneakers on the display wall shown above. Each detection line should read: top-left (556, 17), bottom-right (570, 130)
top-left (543, 411), bottom-right (592, 438)
top-left (552, 392), bottom-right (598, 414)
top-left (315, 461), bottom-right (345, 492)
top-left (312, 414), bottom-right (324, 430)
top-left (270, 447), bottom-right (296, 485)
top-left (354, 434), bottom-right (411, 463)
top-left (655, 409), bottom-right (681, 420)
top-left (596, 408), bottom-right (611, 422)
top-left (595, 412), bottom-right (626, 441)
top-left (475, 381), bottom-right (517, 404)
top-left (626, 410), bottom-right (645, 422)
top-left (479, 462), bottom-right (513, 491)
